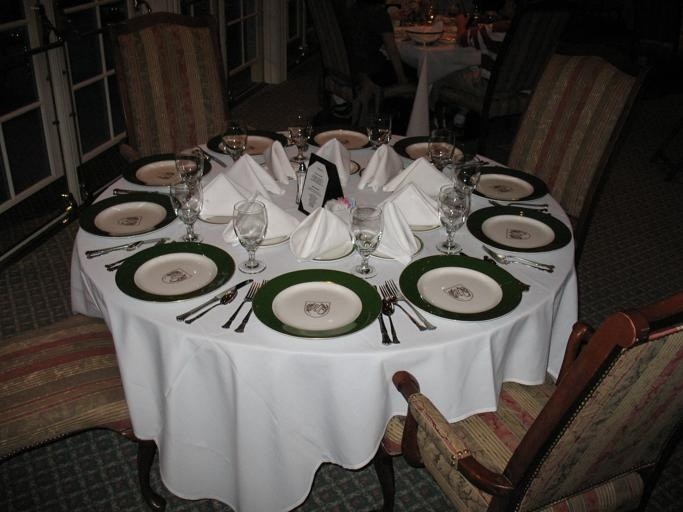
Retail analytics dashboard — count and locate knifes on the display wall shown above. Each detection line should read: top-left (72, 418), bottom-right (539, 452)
top-left (176, 279), bottom-right (254, 326)
top-left (85, 237), bottom-right (171, 256)
top-left (458, 251), bottom-right (531, 292)
top-left (195, 143), bottom-right (228, 170)
top-left (374, 285), bottom-right (391, 343)
top-left (114, 188), bottom-right (169, 198)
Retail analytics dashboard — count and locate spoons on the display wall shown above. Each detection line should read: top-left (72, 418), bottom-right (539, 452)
top-left (381, 297), bottom-right (400, 344)
top-left (86, 241), bottom-right (144, 260)
top-left (185, 290), bottom-right (239, 324)
top-left (484, 256), bottom-right (530, 290)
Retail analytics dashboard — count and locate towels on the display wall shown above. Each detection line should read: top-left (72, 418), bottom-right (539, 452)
top-left (382, 157), bottom-right (454, 195)
top-left (264, 140), bottom-right (297, 184)
top-left (370, 202), bottom-right (420, 265)
top-left (359, 144), bottom-right (404, 190)
top-left (304, 138), bottom-right (351, 190)
top-left (222, 191), bottom-right (301, 247)
top-left (226, 153), bottom-right (285, 200)
top-left (187, 172), bottom-right (249, 217)
top-left (373, 184), bottom-right (445, 229)
top-left (290, 207), bottom-right (353, 260)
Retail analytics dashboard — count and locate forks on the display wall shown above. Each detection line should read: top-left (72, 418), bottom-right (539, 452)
top-left (455, 160), bottom-right (490, 167)
top-left (105, 238), bottom-right (167, 268)
top-left (482, 242), bottom-right (555, 273)
top-left (234, 280), bottom-right (270, 333)
top-left (379, 277), bottom-right (426, 331)
top-left (488, 199), bottom-right (548, 207)
top-left (221, 282), bottom-right (260, 328)
top-left (107, 240), bottom-right (176, 272)
top-left (385, 279), bottom-right (437, 330)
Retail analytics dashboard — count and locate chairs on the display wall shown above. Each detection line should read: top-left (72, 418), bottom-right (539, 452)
top-left (106, 11), bottom-right (235, 169)
top-left (302, 2), bottom-right (389, 128)
top-left (372, 291), bottom-right (682, 507)
top-left (2, 313), bottom-right (173, 511)
top-left (504, 31), bottom-right (649, 275)
top-left (431, 22), bottom-right (562, 160)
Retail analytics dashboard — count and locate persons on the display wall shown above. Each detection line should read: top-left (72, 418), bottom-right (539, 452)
top-left (325, 0), bottom-right (416, 87)
top-left (446, 5), bottom-right (508, 125)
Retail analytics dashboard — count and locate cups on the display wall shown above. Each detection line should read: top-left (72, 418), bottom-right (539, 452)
top-left (222, 118), bottom-right (248, 163)
top-left (233, 199), bottom-right (268, 274)
top-left (367, 114), bottom-right (392, 147)
top-left (428, 129), bottom-right (455, 172)
top-left (175, 151), bottom-right (205, 177)
top-left (452, 160), bottom-right (481, 200)
top-left (350, 205), bottom-right (383, 278)
top-left (288, 126), bottom-right (312, 163)
top-left (169, 177), bottom-right (203, 242)
top-left (176, 147), bottom-right (203, 188)
top-left (436, 185), bottom-right (471, 255)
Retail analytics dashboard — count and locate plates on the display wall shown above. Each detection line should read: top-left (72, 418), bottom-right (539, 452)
top-left (79, 192), bottom-right (177, 237)
top-left (348, 157), bottom-right (361, 174)
top-left (316, 232), bottom-right (357, 261)
top-left (393, 135), bottom-right (477, 164)
top-left (206, 129), bottom-right (289, 156)
top-left (374, 235), bottom-right (424, 260)
top-left (350, 154), bottom-right (361, 177)
top-left (466, 206), bottom-right (572, 253)
top-left (258, 236), bottom-right (290, 247)
top-left (399, 255), bottom-right (523, 321)
top-left (115, 243), bottom-right (236, 302)
top-left (459, 166), bottom-right (548, 201)
top-left (411, 225), bottom-right (443, 232)
top-left (359, 168), bottom-right (365, 177)
top-left (198, 215), bottom-right (234, 225)
top-left (122, 154), bottom-right (213, 186)
top-left (308, 124), bottom-right (371, 150)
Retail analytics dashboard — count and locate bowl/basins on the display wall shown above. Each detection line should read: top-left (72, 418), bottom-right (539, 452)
top-left (405, 31), bottom-right (444, 45)
